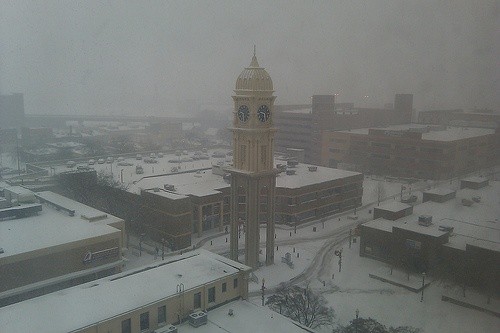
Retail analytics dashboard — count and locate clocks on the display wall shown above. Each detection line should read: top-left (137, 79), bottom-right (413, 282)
top-left (256, 105), bottom-right (270, 123)
top-left (237, 105), bottom-right (250, 123)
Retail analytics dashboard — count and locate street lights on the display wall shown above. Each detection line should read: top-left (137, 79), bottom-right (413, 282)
top-left (420, 272), bottom-right (426, 301)
top-left (260, 277), bottom-right (266, 306)
